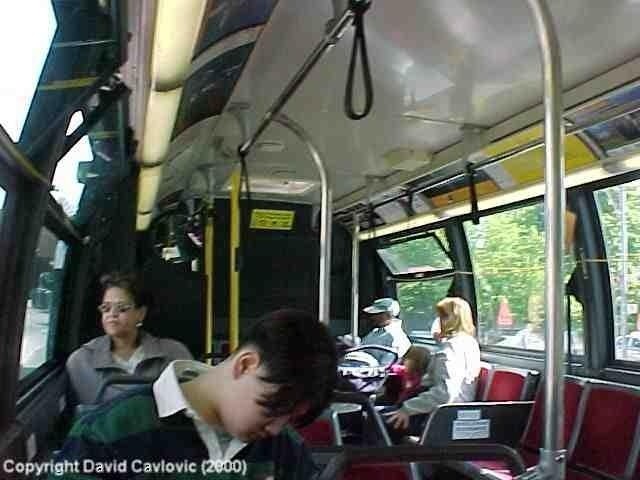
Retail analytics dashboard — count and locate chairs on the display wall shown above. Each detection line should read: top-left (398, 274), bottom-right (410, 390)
top-left (301, 343), bottom-right (640, 479)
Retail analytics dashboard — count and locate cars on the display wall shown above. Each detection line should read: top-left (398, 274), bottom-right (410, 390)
top-left (614, 330), bottom-right (640, 362)
top-left (18, 271), bottom-right (53, 369)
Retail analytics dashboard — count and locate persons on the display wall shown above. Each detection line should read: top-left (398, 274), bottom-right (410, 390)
top-left (47, 271), bottom-right (338, 479)
top-left (359, 296), bottom-right (480, 445)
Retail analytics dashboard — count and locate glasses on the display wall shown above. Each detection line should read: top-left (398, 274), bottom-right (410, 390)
top-left (97, 302), bottom-right (133, 313)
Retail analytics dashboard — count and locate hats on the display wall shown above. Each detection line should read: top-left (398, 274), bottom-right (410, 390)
top-left (362, 297), bottom-right (400, 316)
